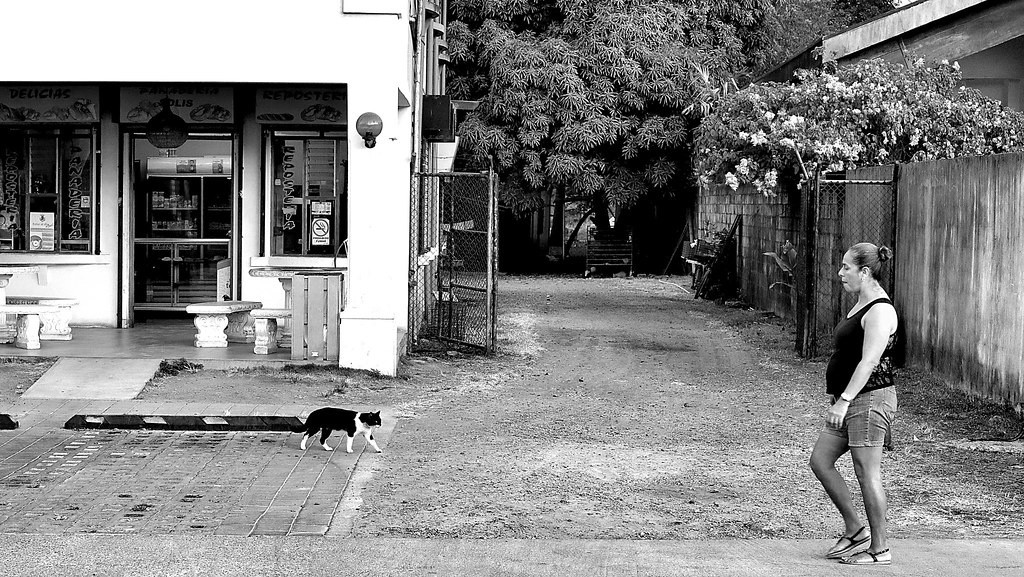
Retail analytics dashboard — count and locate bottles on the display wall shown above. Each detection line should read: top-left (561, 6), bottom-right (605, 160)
top-left (150, 191), bottom-right (200, 251)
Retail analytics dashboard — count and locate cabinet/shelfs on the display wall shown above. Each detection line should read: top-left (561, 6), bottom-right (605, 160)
top-left (150, 177), bottom-right (203, 275)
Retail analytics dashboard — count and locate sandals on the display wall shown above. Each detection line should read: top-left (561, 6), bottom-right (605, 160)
top-left (826, 526), bottom-right (871, 556)
top-left (839, 549), bottom-right (892, 565)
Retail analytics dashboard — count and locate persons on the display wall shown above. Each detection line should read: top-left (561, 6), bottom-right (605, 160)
top-left (809, 243), bottom-right (900, 566)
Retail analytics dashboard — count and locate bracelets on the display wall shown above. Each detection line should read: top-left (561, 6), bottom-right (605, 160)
top-left (841, 393), bottom-right (853, 402)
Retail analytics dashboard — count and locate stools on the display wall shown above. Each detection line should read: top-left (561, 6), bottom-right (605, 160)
top-left (0, 304), bottom-right (60, 350)
top-left (250, 308), bottom-right (294, 356)
top-left (5, 294), bottom-right (81, 342)
top-left (185, 300), bottom-right (263, 349)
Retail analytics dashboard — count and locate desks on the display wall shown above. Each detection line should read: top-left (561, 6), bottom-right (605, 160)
top-left (0, 264), bottom-right (40, 344)
top-left (249, 267), bottom-right (348, 348)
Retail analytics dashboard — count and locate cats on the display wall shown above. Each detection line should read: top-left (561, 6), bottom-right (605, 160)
top-left (290, 407), bottom-right (382, 453)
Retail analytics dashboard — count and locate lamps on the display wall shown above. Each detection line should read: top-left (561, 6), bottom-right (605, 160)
top-left (355, 112), bottom-right (384, 149)
top-left (145, 98), bottom-right (190, 159)
